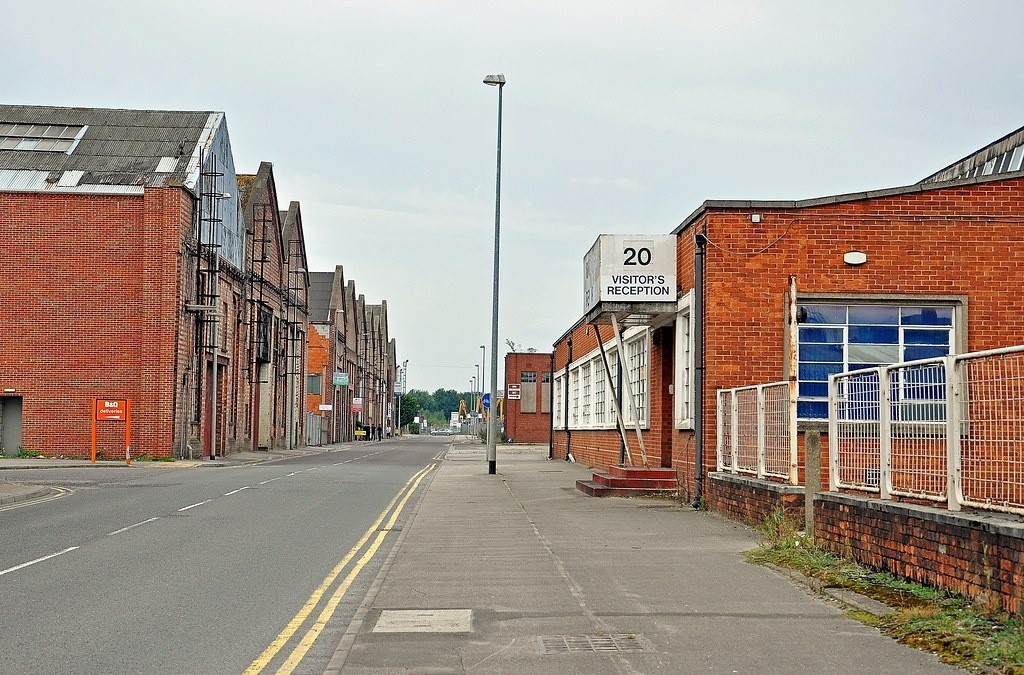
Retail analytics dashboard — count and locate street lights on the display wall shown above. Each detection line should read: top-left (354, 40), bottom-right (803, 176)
top-left (333, 310), bottom-right (344, 444)
top-left (469, 380), bottom-right (473, 411)
top-left (474, 364), bottom-right (480, 397)
top-left (479, 345), bottom-right (486, 395)
top-left (289, 267), bottom-right (306, 453)
top-left (472, 376), bottom-right (477, 406)
top-left (210, 191), bottom-right (231, 459)
top-left (483, 73), bottom-right (506, 475)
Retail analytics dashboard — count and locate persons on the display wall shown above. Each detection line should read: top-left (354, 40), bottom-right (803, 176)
top-left (370, 424), bottom-right (376, 442)
top-left (377, 423), bottom-right (383, 442)
top-left (386, 425), bottom-right (391, 439)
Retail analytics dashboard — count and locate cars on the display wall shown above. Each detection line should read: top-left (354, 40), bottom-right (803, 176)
top-left (430, 428), bottom-right (454, 436)
